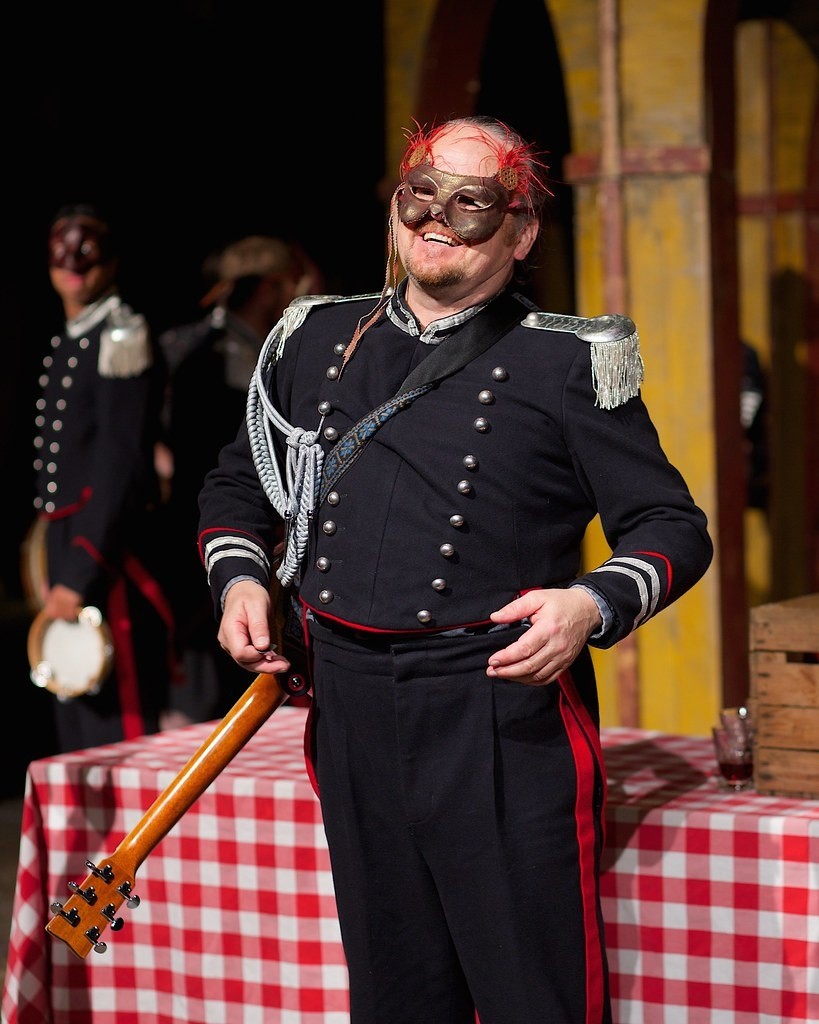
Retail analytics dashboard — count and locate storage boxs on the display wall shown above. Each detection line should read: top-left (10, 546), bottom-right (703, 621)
top-left (746, 594), bottom-right (819, 799)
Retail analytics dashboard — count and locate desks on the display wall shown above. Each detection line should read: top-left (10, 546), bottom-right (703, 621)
top-left (4, 702), bottom-right (819, 1024)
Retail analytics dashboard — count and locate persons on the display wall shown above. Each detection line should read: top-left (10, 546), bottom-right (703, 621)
top-left (195, 119), bottom-right (713, 1023)
top-left (19, 200), bottom-right (768, 752)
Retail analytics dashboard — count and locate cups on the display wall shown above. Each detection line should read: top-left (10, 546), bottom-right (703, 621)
top-left (719, 709), bottom-right (747, 729)
top-left (713, 725), bottom-right (753, 792)
